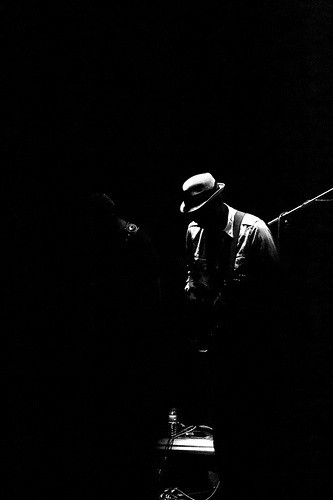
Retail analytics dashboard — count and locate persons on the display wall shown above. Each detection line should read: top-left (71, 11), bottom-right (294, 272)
top-left (55, 192), bottom-right (163, 467)
top-left (178, 170), bottom-right (284, 466)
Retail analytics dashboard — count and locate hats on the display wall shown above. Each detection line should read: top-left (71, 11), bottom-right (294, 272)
top-left (180, 172), bottom-right (225, 213)
